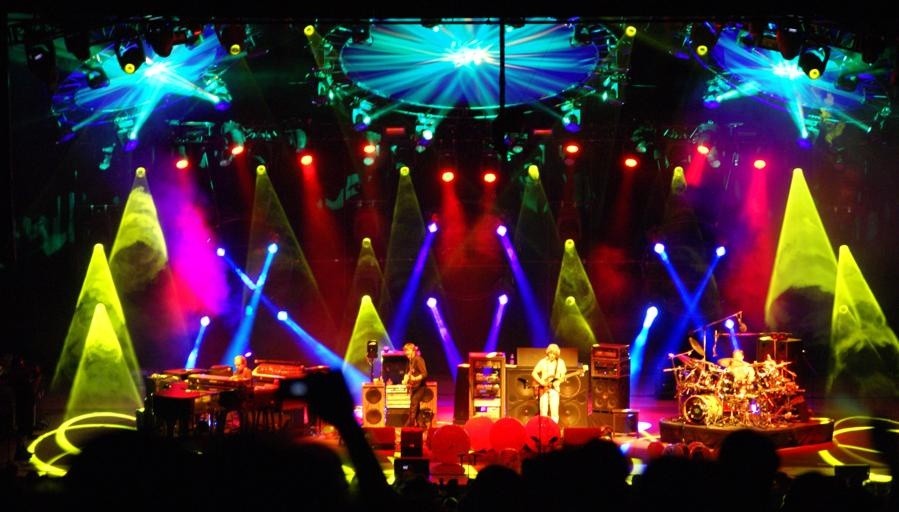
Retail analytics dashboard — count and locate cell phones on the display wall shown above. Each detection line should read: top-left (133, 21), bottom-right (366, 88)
top-left (280, 376), bottom-right (309, 401)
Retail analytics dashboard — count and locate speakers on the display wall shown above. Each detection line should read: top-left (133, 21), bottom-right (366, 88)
top-left (591, 344), bottom-right (630, 412)
top-left (401, 427), bottom-right (423, 456)
top-left (415, 382), bottom-right (436, 427)
top-left (382, 352), bottom-right (409, 384)
top-left (362, 382), bottom-right (386, 428)
top-left (590, 412), bottom-right (638, 435)
top-left (507, 367), bottom-right (539, 423)
top-left (394, 458), bottom-right (429, 479)
top-left (559, 367), bottom-right (588, 428)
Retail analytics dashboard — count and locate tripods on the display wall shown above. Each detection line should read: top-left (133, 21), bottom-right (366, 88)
top-left (718, 384), bottom-right (743, 426)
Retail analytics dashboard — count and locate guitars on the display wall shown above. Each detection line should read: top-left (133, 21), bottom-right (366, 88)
top-left (406, 348), bottom-right (413, 395)
top-left (533, 366), bottom-right (584, 399)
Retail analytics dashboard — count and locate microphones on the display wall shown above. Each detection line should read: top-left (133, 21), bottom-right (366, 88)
top-left (224, 368), bottom-right (231, 374)
top-left (738, 319), bottom-right (747, 334)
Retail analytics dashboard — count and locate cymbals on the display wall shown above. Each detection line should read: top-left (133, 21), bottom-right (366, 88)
top-left (718, 358), bottom-right (741, 369)
top-left (777, 362), bottom-right (791, 367)
top-left (689, 337), bottom-right (705, 356)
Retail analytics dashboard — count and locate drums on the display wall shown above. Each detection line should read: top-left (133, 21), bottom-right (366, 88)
top-left (713, 371), bottom-right (734, 396)
top-left (736, 381), bottom-right (758, 398)
top-left (760, 369), bottom-right (785, 389)
top-left (684, 395), bottom-right (723, 424)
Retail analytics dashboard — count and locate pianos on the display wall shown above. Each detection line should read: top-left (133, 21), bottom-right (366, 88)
top-left (153, 372), bottom-right (249, 437)
